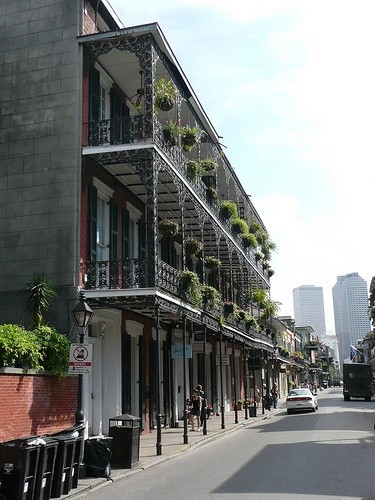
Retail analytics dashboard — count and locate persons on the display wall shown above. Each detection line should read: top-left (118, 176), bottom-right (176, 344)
top-left (299, 379), bottom-right (309, 388)
top-left (187, 385), bottom-right (208, 432)
top-left (271, 384), bottom-right (278, 406)
top-left (261, 384), bottom-right (267, 401)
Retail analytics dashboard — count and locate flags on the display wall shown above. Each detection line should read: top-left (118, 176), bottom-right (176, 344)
top-left (351, 347), bottom-right (360, 357)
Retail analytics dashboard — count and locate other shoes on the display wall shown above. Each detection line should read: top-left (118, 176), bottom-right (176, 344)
top-left (190, 429), bottom-right (194, 431)
top-left (197, 429), bottom-right (200, 431)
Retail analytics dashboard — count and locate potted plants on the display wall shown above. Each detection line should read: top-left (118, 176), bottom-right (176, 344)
top-left (156, 79), bottom-right (175, 111)
top-left (235, 308), bottom-right (247, 321)
top-left (256, 253), bottom-right (262, 260)
top-left (205, 257), bottom-right (222, 269)
top-left (224, 302), bottom-right (237, 314)
top-left (231, 219), bottom-right (250, 233)
top-left (185, 239), bottom-right (202, 255)
top-left (257, 232), bottom-right (268, 244)
top-left (263, 262), bottom-right (270, 269)
top-left (243, 234), bottom-right (257, 248)
top-left (220, 200), bottom-right (238, 219)
top-left (250, 223), bottom-right (261, 233)
top-left (268, 270), bottom-right (274, 276)
top-left (159, 220), bottom-right (178, 237)
top-left (181, 127), bottom-right (205, 152)
top-left (163, 119), bottom-right (177, 140)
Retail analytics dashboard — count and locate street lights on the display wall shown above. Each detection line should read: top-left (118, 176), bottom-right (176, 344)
top-left (71, 291), bottom-right (96, 478)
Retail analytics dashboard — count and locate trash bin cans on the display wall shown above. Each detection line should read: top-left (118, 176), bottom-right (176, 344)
top-left (0, 423), bottom-right (85, 500)
top-left (248, 407), bottom-right (258, 417)
top-left (108, 414), bottom-right (143, 470)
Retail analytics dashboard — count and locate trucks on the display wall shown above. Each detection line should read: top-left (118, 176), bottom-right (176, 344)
top-left (341, 362), bottom-right (374, 402)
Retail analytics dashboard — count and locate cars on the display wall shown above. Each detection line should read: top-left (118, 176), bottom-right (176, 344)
top-left (334, 381), bottom-right (341, 387)
top-left (285, 388), bottom-right (319, 414)
